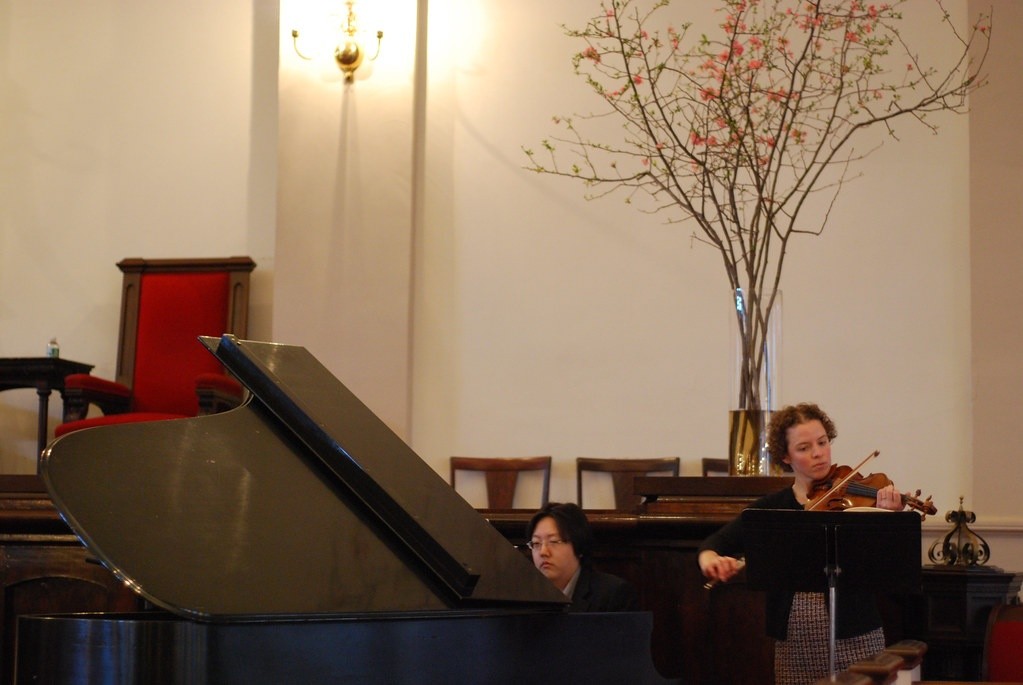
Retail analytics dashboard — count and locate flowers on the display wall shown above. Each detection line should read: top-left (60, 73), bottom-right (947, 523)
top-left (520, 1), bottom-right (994, 479)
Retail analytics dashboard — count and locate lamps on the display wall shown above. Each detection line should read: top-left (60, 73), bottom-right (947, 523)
top-left (291, 1), bottom-right (384, 85)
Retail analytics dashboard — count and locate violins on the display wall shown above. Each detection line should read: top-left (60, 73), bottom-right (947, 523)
top-left (805, 463), bottom-right (937, 523)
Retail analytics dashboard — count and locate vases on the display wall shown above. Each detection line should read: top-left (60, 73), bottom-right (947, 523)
top-left (727, 286), bottom-right (784, 479)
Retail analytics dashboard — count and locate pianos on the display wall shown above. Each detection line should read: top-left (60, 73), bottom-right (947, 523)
top-left (15, 334), bottom-right (685, 685)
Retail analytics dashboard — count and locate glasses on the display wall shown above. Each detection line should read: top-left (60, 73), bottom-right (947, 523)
top-left (527, 536), bottom-right (573, 550)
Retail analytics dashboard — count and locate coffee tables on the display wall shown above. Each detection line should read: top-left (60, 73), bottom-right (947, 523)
top-left (0, 357), bottom-right (97, 499)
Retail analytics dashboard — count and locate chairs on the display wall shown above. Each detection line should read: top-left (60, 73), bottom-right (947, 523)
top-left (53, 256), bottom-right (257, 442)
top-left (576, 456), bottom-right (681, 514)
top-left (448, 456), bottom-right (553, 513)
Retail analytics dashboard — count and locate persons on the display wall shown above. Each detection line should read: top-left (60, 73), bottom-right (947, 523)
top-left (698, 404), bottom-right (910, 685)
top-left (528, 502), bottom-right (639, 611)
